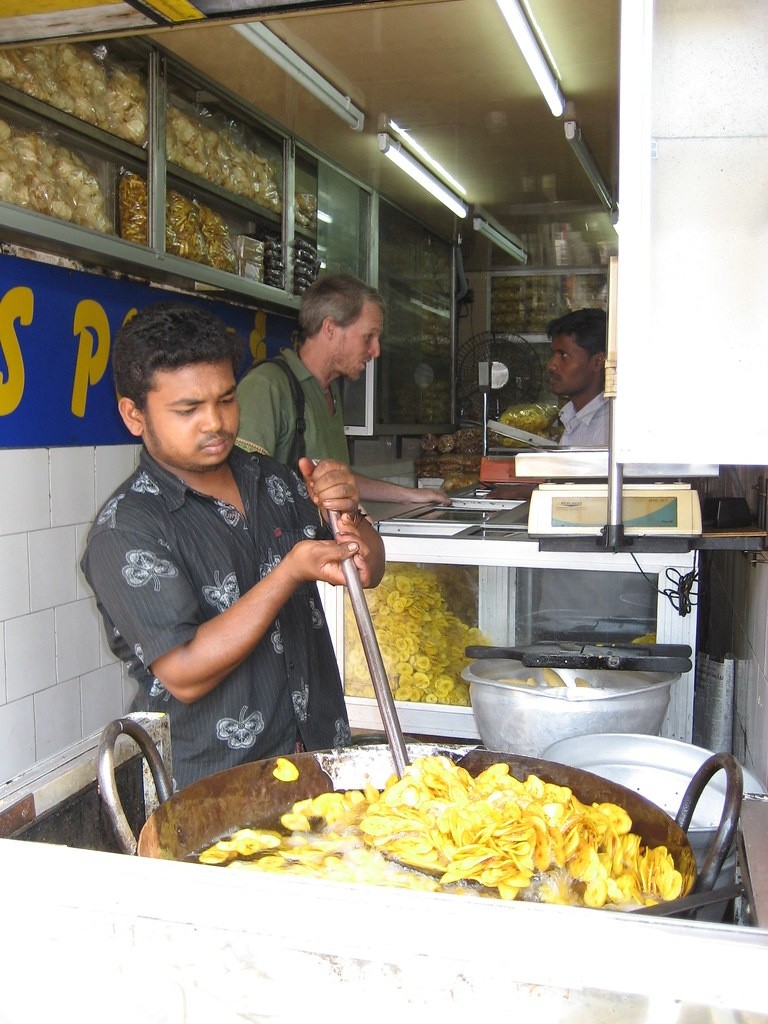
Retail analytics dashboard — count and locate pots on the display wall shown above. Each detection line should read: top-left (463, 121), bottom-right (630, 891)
top-left (459, 645), bottom-right (683, 759)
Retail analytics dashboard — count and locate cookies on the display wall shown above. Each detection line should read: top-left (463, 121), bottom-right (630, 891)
top-left (414, 429), bottom-right (485, 453)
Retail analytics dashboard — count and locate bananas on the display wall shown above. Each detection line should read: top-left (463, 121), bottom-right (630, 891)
top-left (494, 665), bottom-right (598, 688)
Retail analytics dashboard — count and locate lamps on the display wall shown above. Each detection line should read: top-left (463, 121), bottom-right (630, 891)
top-left (472, 217), bottom-right (528, 265)
top-left (473, 202), bottom-right (528, 253)
top-left (496, 0), bottom-right (566, 121)
top-left (231, 21), bottom-right (365, 135)
top-left (377, 134), bottom-right (469, 220)
top-left (378, 113), bottom-right (468, 199)
top-left (563, 121), bottom-right (613, 210)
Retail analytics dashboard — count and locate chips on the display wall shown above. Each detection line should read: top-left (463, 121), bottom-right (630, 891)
top-left (0, 43), bottom-right (318, 233)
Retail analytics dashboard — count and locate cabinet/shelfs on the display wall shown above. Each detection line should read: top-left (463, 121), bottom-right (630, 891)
top-left (0, 34), bottom-right (768, 516)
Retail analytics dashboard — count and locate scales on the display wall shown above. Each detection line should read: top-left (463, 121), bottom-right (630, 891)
top-left (477, 361), bottom-right (553, 487)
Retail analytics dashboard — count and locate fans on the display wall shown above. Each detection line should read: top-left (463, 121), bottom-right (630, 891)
top-left (455, 330), bottom-right (542, 457)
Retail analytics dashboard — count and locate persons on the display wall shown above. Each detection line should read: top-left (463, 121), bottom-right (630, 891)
top-left (546, 305), bottom-right (611, 448)
top-left (79, 306), bottom-right (384, 793)
top-left (233, 274), bottom-right (452, 509)
